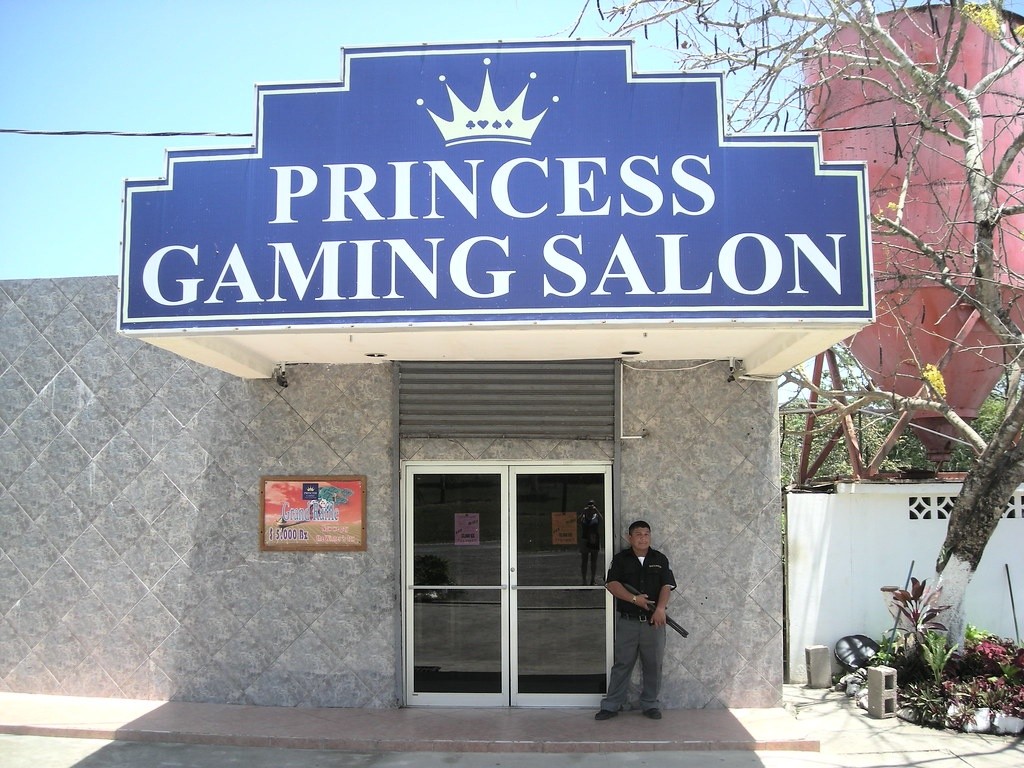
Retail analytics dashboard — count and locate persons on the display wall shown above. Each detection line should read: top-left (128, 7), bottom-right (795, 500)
top-left (595, 520), bottom-right (677, 720)
top-left (578, 500), bottom-right (603, 586)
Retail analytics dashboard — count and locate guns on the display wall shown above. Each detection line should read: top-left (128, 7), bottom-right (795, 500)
top-left (604, 580), bottom-right (689, 638)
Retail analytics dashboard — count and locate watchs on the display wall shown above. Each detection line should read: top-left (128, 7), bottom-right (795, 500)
top-left (631, 596), bottom-right (636, 603)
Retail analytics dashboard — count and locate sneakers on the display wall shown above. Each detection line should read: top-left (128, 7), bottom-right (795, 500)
top-left (595, 710), bottom-right (618, 720)
top-left (642, 708), bottom-right (661, 719)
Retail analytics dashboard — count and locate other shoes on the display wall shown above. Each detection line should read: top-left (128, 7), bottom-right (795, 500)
top-left (590, 582), bottom-right (598, 585)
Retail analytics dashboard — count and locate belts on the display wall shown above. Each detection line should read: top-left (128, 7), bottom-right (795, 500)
top-left (621, 613), bottom-right (650, 622)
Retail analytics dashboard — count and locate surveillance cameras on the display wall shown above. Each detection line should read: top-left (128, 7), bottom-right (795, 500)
top-left (276, 375), bottom-right (288, 388)
top-left (727, 375), bottom-right (735, 382)
